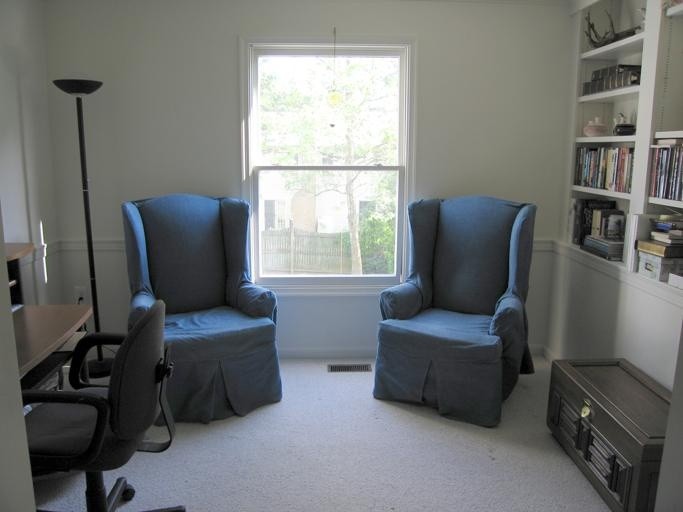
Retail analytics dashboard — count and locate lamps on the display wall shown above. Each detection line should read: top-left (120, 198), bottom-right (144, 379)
top-left (52, 80), bottom-right (116, 377)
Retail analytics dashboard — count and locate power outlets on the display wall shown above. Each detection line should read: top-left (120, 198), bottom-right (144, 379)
top-left (73, 286), bottom-right (86, 307)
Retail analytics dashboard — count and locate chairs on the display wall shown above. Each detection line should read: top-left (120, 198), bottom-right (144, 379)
top-left (373, 196), bottom-right (536, 428)
top-left (121, 193), bottom-right (282, 426)
top-left (20, 300), bottom-right (187, 512)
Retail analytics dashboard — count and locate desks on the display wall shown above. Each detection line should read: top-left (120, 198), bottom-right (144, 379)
top-left (12, 303), bottom-right (93, 415)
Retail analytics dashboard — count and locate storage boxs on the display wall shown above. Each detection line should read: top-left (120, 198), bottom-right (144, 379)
top-left (638, 251), bottom-right (683, 284)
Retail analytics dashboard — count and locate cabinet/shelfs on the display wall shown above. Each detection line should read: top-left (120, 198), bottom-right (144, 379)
top-left (548, 0), bottom-right (683, 294)
top-left (546, 357), bottom-right (673, 511)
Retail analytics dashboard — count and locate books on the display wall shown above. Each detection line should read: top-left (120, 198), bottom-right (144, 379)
top-left (566, 138), bottom-right (683, 289)
